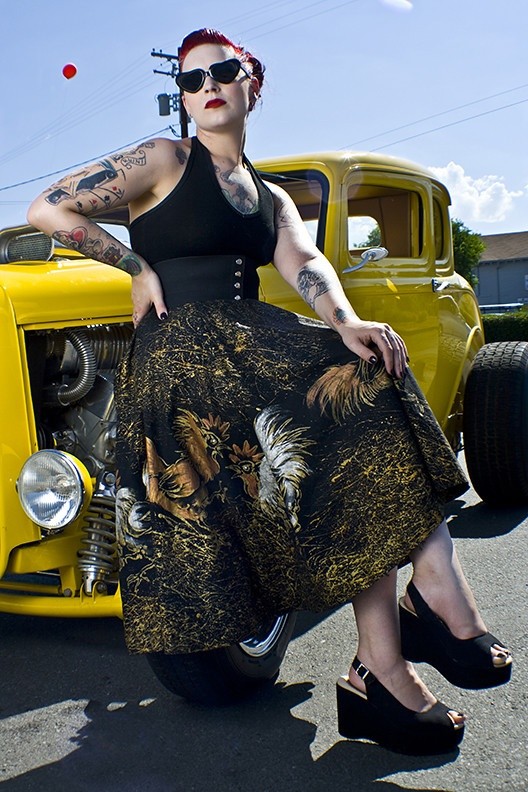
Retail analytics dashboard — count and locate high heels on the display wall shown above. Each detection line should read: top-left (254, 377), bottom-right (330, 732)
top-left (336, 655), bottom-right (467, 757)
top-left (398, 579), bottom-right (513, 691)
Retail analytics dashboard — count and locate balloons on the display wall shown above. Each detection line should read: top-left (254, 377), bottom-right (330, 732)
top-left (62, 64), bottom-right (77, 79)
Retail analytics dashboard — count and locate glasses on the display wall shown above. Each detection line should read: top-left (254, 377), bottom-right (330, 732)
top-left (175, 57), bottom-right (251, 94)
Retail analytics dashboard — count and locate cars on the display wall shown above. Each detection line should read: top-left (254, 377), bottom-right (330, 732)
top-left (0, 151), bottom-right (528, 620)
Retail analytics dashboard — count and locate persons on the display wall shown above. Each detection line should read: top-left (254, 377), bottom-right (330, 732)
top-left (27, 30), bottom-right (511, 754)
